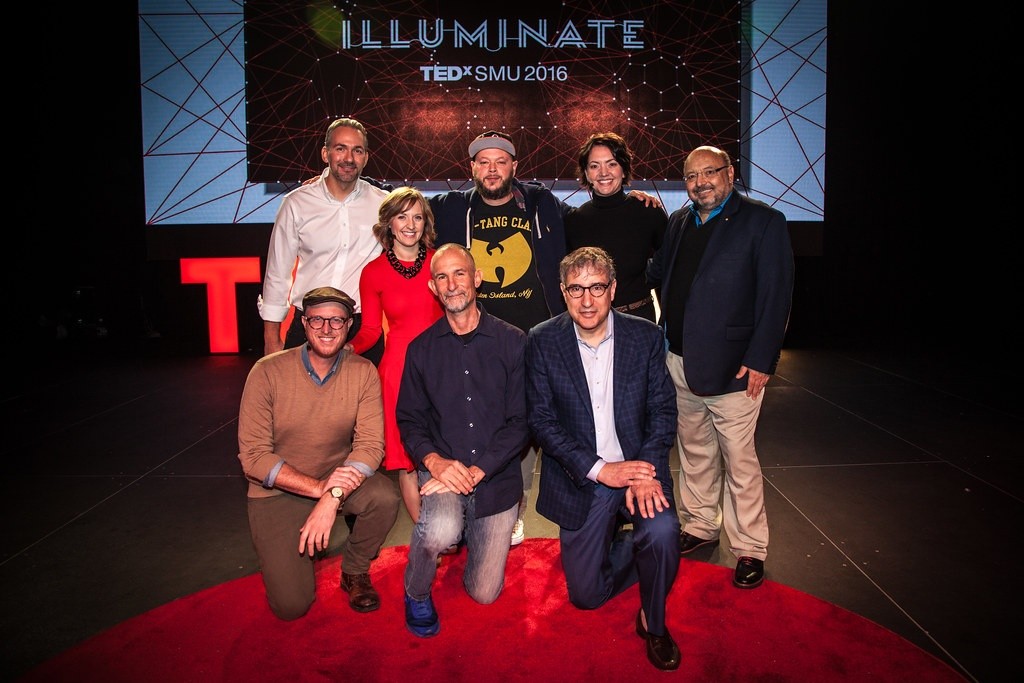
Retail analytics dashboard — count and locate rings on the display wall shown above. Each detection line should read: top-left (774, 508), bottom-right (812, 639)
top-left (652, 492), bottom-right (658, 497)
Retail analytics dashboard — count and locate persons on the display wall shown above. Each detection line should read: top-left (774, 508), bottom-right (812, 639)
top-left (565, 132), bottom-right (670, 322)
top-left (238, 286), bottom-right (400, 622)
top-left (342, 186), bottom-right (445, 524)
top-left (395, 244), bottom-right (528, 637)
top-left (659, 146), bottom-right (794, 588)
top-left (258, 119), bottom-right (393, 367)
top-left (529, 246), bottom-right (681, 671)
top-left (302, 131), bottom-right (661, 545)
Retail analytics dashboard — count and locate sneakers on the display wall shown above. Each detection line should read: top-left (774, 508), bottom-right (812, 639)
top-left (404, 593), bottom-right (441, 637)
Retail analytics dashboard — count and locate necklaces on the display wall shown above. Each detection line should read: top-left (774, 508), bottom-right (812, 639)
top-left (386, 240), bottom-right (426, 279)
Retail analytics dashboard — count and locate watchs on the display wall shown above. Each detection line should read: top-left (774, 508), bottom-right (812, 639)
top-left (327, 487), bottom-right (344, 509)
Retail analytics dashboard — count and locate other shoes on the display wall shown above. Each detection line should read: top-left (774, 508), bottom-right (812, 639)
top-left (510, 519), bottom-right (524, 545)
top-left (442, 545), bottom-right (457, 553)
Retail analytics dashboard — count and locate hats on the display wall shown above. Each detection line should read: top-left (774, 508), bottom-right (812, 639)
top-left (302, 287), bottom-right (356, 313)
top-left (469, 131), bottom-right (515, 159)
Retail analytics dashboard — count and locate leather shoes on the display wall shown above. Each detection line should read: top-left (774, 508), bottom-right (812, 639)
top-left (679, 531), bottom-right (719, 554)
top-left (734, 557), bottom-right (765, 588)
top-left (637, 609), bottom-right (680, 670)
top-left (341, 573), bottom-right (379, 612)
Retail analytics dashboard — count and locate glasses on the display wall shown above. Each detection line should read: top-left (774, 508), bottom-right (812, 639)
top-left (683, 165), bottom-right (729, 183)
top-left (307, 315), bottom-right (349, 330)
top-left (566, 280), bottom-right (611, 299)
